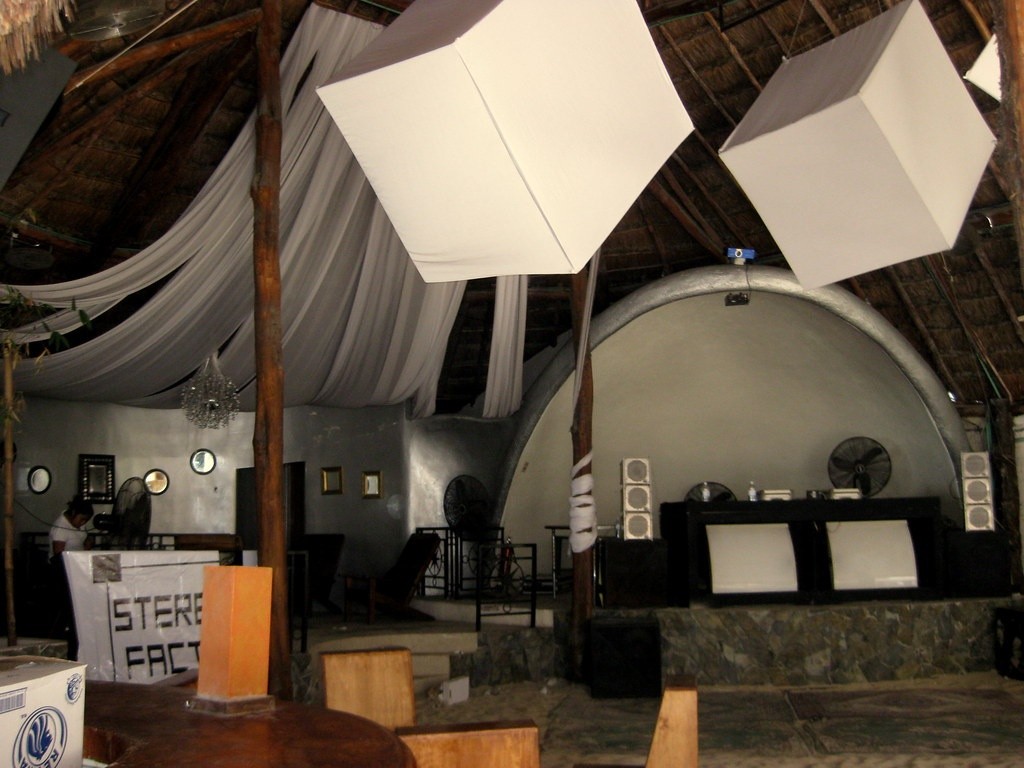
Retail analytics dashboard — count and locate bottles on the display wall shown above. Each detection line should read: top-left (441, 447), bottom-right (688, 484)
top-left (748, 480), bottom-right (757, 501)
top-left (702, 482), bottom-right (710, 502)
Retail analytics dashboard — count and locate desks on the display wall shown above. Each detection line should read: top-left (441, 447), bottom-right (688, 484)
top-left (0, 679), bottom-right (414, 768)
top-left (661, 496), bottom-right (949, 605)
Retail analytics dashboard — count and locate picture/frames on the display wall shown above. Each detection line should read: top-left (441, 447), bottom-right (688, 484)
top-left (320, 467), bottom-right (344, 494)
top-left (362, 471), bottom-right (382, 499)
top-left (78, 453), bottom-right (115, 504)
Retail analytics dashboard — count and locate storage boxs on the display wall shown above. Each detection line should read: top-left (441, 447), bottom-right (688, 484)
top-left (0, 650), bottom-right (85, 768)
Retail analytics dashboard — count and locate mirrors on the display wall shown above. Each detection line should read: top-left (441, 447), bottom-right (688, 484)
top-left (143, 469), bottom-right (169, 496)
top-left (28, 465), bottom-right (51, 494)
top-left (190, 449), bottom-right (216, 475)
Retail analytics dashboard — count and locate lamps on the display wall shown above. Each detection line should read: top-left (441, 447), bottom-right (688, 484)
top-left (180, 349), bottom-right (241, 428)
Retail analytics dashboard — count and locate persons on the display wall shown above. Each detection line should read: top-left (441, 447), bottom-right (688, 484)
top-left (48, 495), bottom-right (96, 638)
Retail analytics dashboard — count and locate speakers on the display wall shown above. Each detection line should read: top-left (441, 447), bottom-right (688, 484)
top-left (619, 458), bottom-right (653, 542)
top-left (960, 451), bottom-right (994, 531)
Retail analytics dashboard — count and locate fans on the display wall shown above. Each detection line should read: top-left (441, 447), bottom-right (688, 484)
top-left (827, 436), bottom-right (891, 499)
top-left (685, 482), bottom-right (735, 502)
top-left (443, 475), bottom-right (494, 543)
top-left (91, 478), bottom-right (151, 552)
